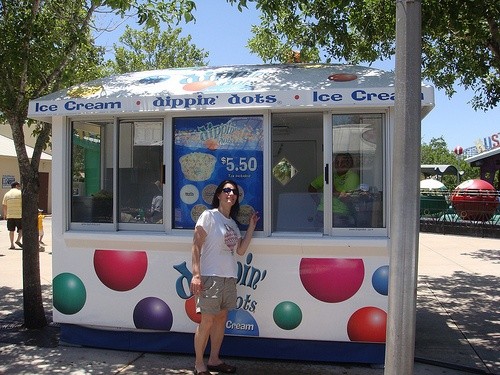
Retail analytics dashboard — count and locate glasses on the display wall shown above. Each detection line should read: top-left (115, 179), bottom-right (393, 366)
top-left (221, 188), bottom-right (238, 194)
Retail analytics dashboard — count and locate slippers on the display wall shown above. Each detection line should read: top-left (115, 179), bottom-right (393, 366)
top-left (194, 367), bottom-right (212, 375)
top-left (207, 360), bottom-right (237, 373)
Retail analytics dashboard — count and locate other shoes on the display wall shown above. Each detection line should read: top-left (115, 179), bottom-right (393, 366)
top-left (10, 246), bottom-right (14, 249)
top-left (15, 241), bottom-right (23, 247)
top-left (40, 241), bottom-right (44, 245)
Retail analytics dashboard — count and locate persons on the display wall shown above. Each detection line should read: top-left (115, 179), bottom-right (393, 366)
top-left (189, 179), bottom-right (261, 375)
top-left (145, 179), bottom-right (163, 224)
top-left (38, 209), bottom-right (51, 246)
top-left (308, 153), bottom-right (360, 232)
top-left (2, 181), bottom-right (23, 250)
